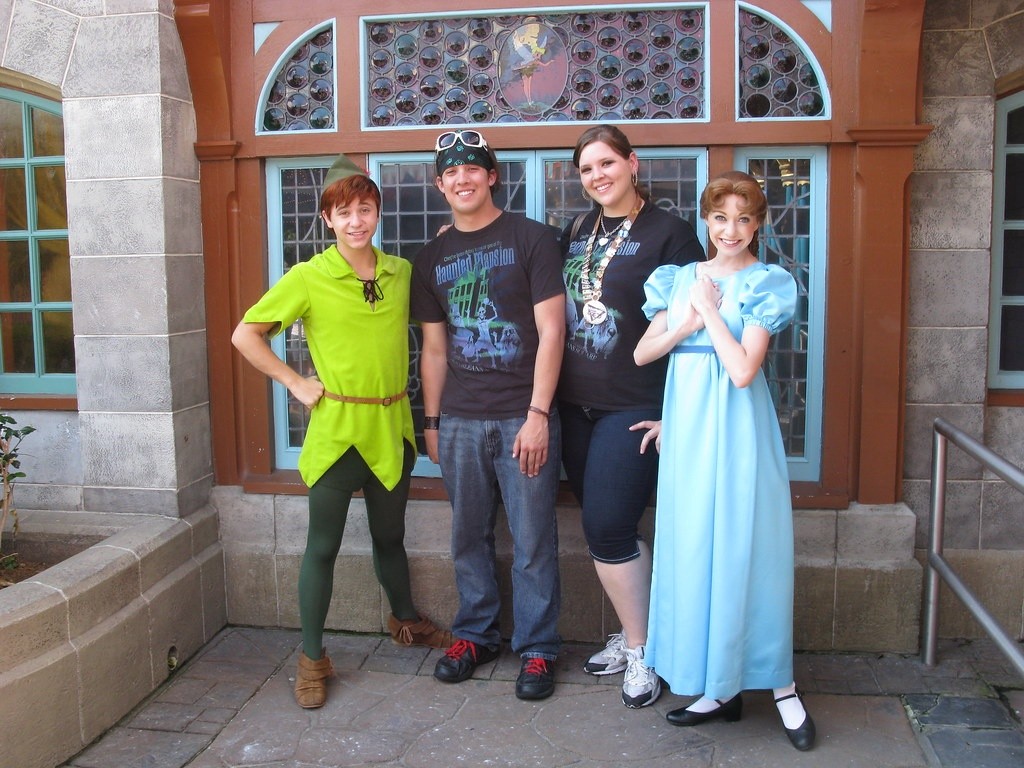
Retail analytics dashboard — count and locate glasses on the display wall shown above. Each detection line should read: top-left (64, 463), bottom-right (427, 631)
top-left (436, 129), bottom-right (494, 163)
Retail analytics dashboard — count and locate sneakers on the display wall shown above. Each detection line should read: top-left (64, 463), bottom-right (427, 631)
top-left (434, 641), bottom-right (498, 682)
top-left (388, 612), bottom-right (462, 649)
top-left (622, 644), bottom-right (660, 708)
top-left (515, 656), bottom-right (558, 698)
top-left (295, 647), bottom-right (332, 708)
top-left (584, 628), bottom-right (630, 676)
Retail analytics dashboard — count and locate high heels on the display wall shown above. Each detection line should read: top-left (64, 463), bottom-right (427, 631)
top-left (772, 688), bottom-right (816, 751)
top-left (667, 691), bottom-right (744, 725)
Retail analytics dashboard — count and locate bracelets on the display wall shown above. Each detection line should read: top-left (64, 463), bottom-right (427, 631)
top-left (528, 406), bottom-right (549, 417)
top-left (424, 415), bottom-right (439, 429)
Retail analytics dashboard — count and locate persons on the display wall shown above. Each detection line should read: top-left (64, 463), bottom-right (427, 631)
top-left (633, 172), bottom-right (817, 751)
top-left (230, 154), bottom-right (460, 708)
top-left (435, 126), bottom-right (707, 705)
top-left (410, 130), bottom-right (567, 699)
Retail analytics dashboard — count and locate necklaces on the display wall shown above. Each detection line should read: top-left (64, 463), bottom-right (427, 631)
top-left (582, 192), bottom-right (645, 329)
top-left (599, 210), bottom-right (628, 246)
